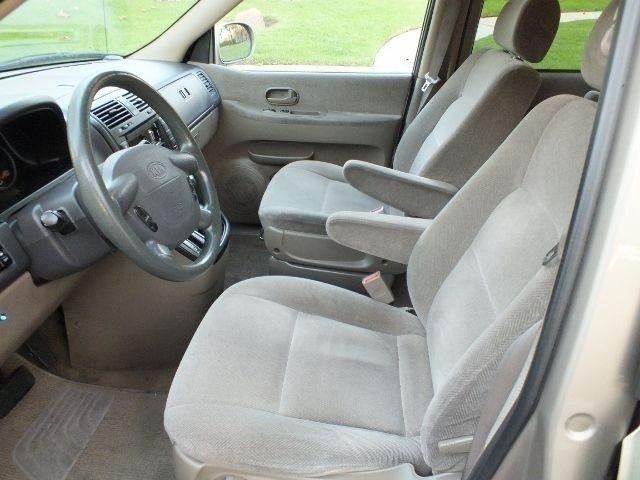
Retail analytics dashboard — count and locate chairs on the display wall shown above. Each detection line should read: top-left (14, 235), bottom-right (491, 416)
top-left (163, 0), bottom-right (626, 478)
top-left (256, 2), bottom-right (558, 296)
